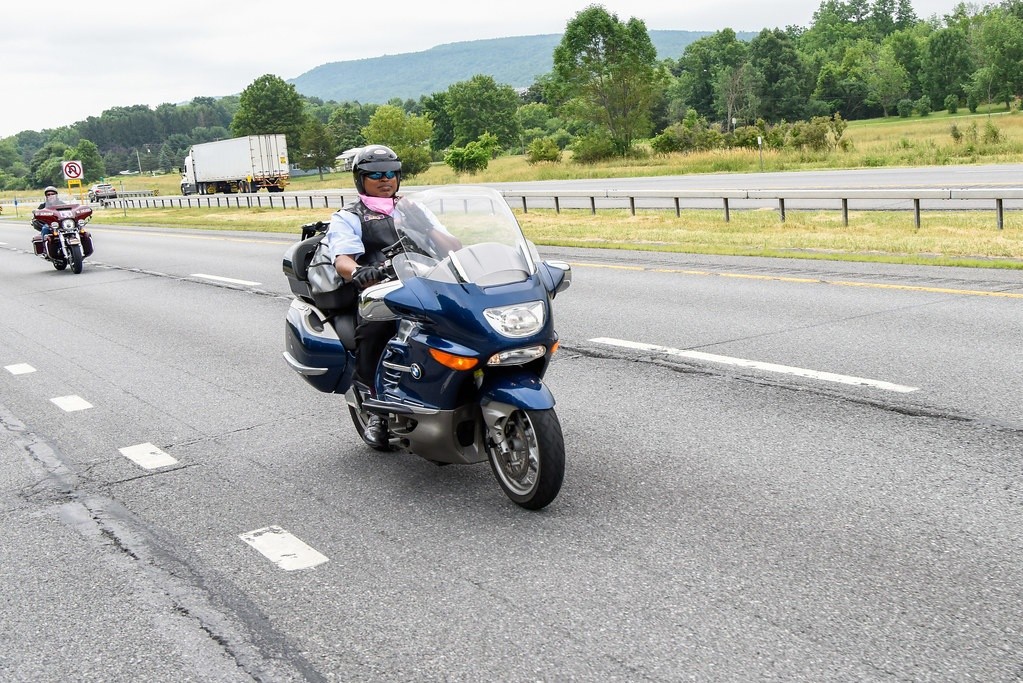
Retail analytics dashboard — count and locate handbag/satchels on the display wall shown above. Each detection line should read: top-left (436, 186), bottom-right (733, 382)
top-left (307, 236), bottom-right (343, 293)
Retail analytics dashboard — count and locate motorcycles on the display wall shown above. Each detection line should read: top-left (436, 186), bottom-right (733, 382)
top-left (280, 183), bottom-right (583, 511)
top-left (30, 193), bottom-right (95, 275)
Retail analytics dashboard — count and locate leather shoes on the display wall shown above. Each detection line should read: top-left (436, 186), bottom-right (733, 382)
top-left (365, 415), bottom-right (389, 446)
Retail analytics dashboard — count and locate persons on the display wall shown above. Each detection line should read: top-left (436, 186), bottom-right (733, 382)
top-left (31, 185), bottom-right (71, 259)
top-left (326, 143), bottom-right (463, 447)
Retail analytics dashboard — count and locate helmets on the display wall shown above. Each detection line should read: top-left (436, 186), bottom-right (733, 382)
top-left (44, 186), bottom-right (58, 195)
top-left (352, 144), bottom-right (402, 194)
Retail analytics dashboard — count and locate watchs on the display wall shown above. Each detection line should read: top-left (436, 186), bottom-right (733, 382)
top-left (351, 265), bottom-right (361, 277)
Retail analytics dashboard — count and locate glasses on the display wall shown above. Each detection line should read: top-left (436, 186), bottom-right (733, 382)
top-left (46, 193), bottom-right (55, 196)
top-left (364, 170), bottom-right (396, 180)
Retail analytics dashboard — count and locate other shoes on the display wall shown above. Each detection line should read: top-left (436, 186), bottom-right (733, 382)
top-left (43, 253), bottom-right (48, 259)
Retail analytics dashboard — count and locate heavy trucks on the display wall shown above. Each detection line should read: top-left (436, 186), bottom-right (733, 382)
top-left (180, 134), bottom-right (291, 195)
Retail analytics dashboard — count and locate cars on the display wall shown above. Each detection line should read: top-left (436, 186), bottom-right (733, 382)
top-left (87, 183), bottom-right (117, 202)
